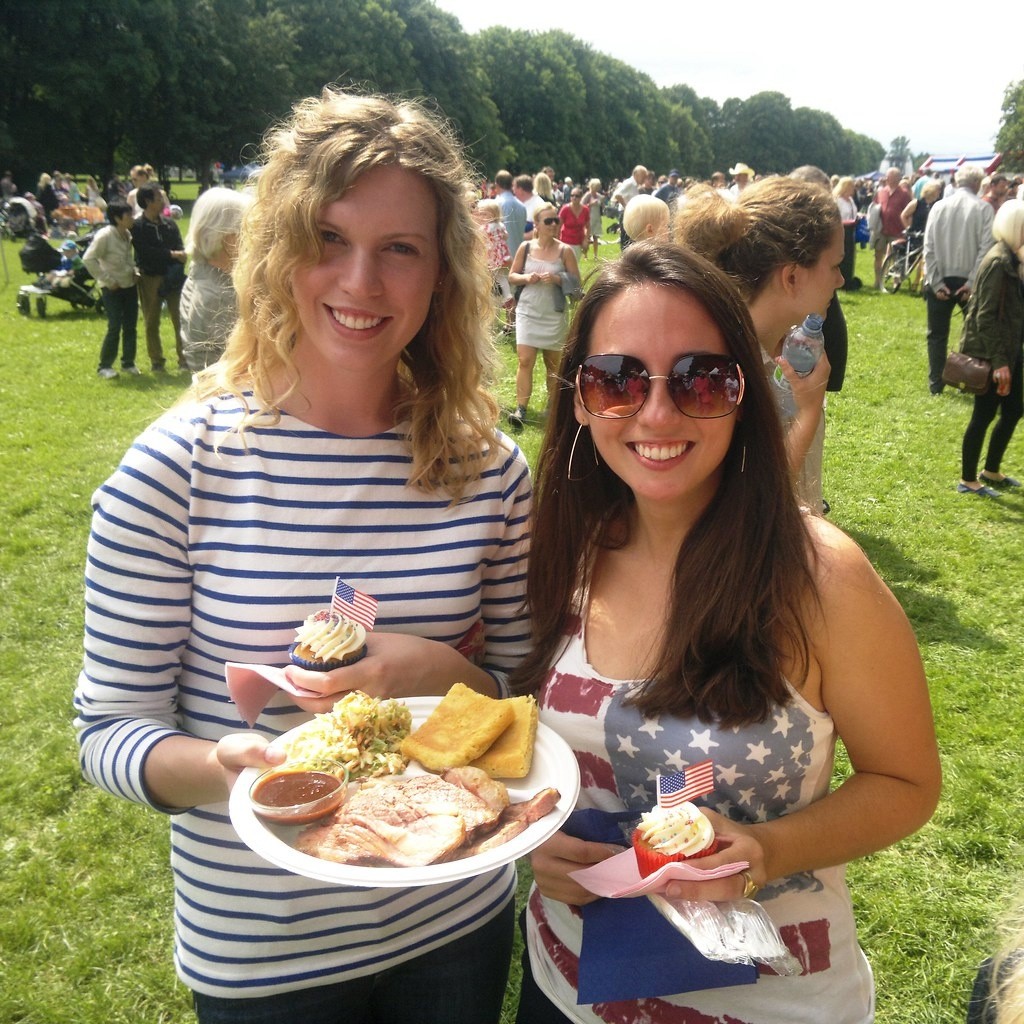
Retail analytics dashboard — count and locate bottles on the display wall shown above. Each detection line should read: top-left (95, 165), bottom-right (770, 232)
top-left (768, 312), bottom-right (825, 419)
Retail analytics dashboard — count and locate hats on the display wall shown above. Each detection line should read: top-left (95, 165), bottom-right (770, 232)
top-left (729, 163), bottom-right (754, 177)
top-left (62, 240), bottom-right (76, 249)
top-left (670, 169), bottom-right (679, 176)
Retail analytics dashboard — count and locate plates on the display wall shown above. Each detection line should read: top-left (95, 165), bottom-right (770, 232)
top-left (228, 695), bottom-right (581, 888)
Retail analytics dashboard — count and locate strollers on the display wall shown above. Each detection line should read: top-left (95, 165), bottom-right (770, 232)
top-left (0, 196), bottom-right (108, 319)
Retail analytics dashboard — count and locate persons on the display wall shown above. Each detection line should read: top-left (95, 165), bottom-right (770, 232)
top-left (462, 162), bottom-right (762, 330)
top-left (179, 187), bottom-right (247, 375)
top-left (678, 177), bottom-right (846, 481)
top-left (923, 164), bottom-right (995, 394)
top-left (0, 164), bottom-right (187, 378)
top-left (580, 370), bottom-right (648, 415)
top-left (508, 203), bottom-right (580, 428)
top-left (518, 240), bottom-right (941, 1024)
top-left (75, 84), bottom-right (536, 1024)
top-left (832, 167), bottom-right (958, 294)
top-left (959, 199), bottom-right (1024, 497)
top-left (979, 170), bottom-right (1024, 212)
top-left (788, 166), bottom-right (849, 514)
top-left (670, 368), bottom-right (740, 413)
top-left (966, 890), bottom-right (1024, 1024)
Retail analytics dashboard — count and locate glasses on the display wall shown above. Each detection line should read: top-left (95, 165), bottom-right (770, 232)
top-left (544, 218), bottom-right (561, 225)
top-left (576, 351), bottom-right (744, 419)
top-left (570, 194), bottom-right (579, 197)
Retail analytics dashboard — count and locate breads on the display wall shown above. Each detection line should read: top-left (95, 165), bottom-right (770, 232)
top-left (399, 682), bottom-right (540, 778)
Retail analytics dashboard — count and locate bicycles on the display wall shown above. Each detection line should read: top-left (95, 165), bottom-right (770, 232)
top-left (879, 227), bottom-right (925, 294)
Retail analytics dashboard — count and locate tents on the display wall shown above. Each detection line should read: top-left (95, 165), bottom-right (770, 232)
top-left (219, 163), bottom-right (262, 186)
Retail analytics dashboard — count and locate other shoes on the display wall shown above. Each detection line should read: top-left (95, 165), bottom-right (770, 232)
top-left (979, 471), bottom-right (1022, 489)
top-left (502, 298), bottom-right (514, 308)
top-left (99, 367), bottom-right (118, 379)
top-left (957, 484), bottom-right (1000, 498)
top-left (507, 414), bottom-right (523, 428)
top-left (121, 367), bottom-right (141, 376)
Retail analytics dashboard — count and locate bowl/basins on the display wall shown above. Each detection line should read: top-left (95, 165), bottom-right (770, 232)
top-left (248, 758), bottom-right (350, 827)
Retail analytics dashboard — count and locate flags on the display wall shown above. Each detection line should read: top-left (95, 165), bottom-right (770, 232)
top-left (660, 758), bottom-right (714, 807)
top-left (334, 578), bottom-right (378, 632)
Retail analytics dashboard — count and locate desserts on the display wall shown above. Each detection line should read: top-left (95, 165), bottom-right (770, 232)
top-left (632, 799), bottom-right (719, 880)
top-left (288, 609), bottom-right (368, 671)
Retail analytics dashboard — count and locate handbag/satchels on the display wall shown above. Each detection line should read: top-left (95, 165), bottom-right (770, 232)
top-left (854, 216), bottom-right (871, 249)
top-left (822, 291), bottom-right (848, 391)
top-left (942, 350), bottom-right (994, 395)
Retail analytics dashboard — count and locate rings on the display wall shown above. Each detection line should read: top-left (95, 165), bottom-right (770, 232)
top-left (741, 871), bottom-right (755, 898)
top-left (996, 373), bottom-right (1000, 378)
